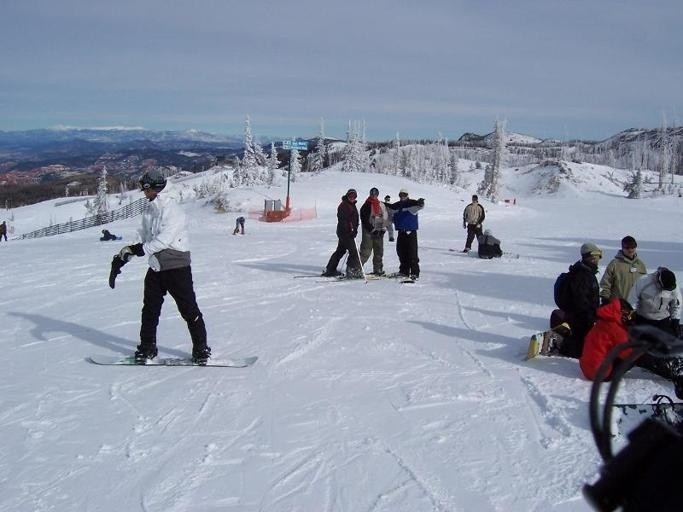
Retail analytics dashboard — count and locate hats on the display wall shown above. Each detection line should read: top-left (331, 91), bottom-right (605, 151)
top-left (580, 243), bottom-right (602, 259)
top-left (347, 187), bottom-right (409, 197)
top-left (622, 236), bottom-right (637, 248)
top-left (657, 267), bottom-right (676, 291)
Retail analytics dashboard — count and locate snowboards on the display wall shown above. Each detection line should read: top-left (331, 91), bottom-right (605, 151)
top-left (600, 402), bottom-right (682, 438)
top-left (449, 248), bottom-right (478, 254)
top-left (527, 322), bottom-right (573, 359)
top-left (89, 353), bottom-right (258, 367)
top-left (468, 251), bottom-right (519, 258)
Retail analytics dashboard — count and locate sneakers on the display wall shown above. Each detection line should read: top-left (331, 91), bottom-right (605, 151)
top-left (192, 346), bottom-right (211, 365)
top-left (135, 343), bottom-right (158, 362)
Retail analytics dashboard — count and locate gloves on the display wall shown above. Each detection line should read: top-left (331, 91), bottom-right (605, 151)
top-left (119, 243), bottom-right (144, 262)
top-left (109, 254), bottom-right (123, 289)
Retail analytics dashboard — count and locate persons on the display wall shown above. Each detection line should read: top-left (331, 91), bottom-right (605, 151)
top-left (527, 235), bottom-right (681, 382)
top-left (383, 189), bottom-right (425, 280)
top-left (462, 195), bottom-right (487, 253)
top-left (384, 196), bottom-right (395, 241)
top-left (234, 217), bottom-right (245, 234)
top-left (360, 188), bottom-right (388, 274)
top-left (100, 230), bottom-right (122, 240)
top-left (326, 189), bottom-right (361, 275)
top-left (478, 229), bottom-right (502, 259)
top-left (109, 171), bottom-right (207, 362)
top-left (0, 221), bottom-right (8, 241)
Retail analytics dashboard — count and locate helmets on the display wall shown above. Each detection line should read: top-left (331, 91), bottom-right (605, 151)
top-left (138, 170), bottom-right (167, 191)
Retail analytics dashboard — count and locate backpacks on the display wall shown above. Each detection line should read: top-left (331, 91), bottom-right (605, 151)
top-left (554, 264), bottom-right (578, 312)
